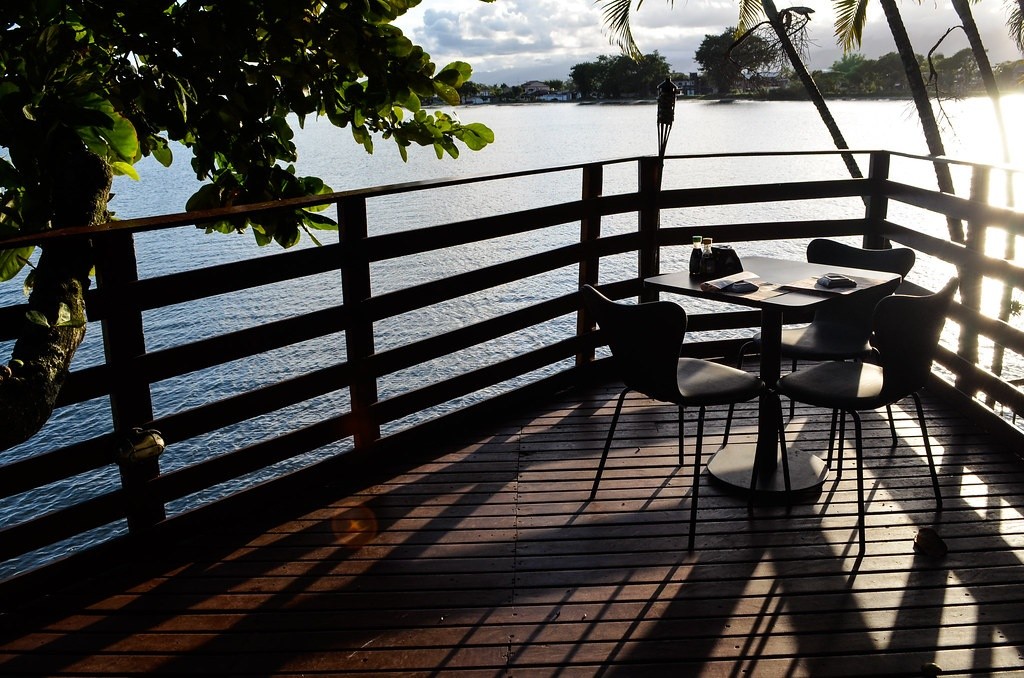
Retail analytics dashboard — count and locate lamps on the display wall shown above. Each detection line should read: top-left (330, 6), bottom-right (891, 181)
top-left (656, 77), bottom-right (677, 156)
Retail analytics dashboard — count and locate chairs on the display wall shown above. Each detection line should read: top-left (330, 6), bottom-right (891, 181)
top-left (723, 238), bottom-right (916, 470)
top-left (747, 278), bottom-right (963, 526)
top-left (583, 284), bottom-right (795, 553)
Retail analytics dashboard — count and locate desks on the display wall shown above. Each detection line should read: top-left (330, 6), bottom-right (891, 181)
top-left (644, 254), bottom-right (904, 500)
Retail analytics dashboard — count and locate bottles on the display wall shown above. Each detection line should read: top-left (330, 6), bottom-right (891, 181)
top-left (702, 237), bottom-right (714, 282)
top-left (689, 236), bottom-right (703, 281)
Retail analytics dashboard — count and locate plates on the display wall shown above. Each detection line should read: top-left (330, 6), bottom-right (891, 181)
top-left (817, 275), bottom-right (857, 289)
top-left (730, 281), bottom-right (759, 294)
